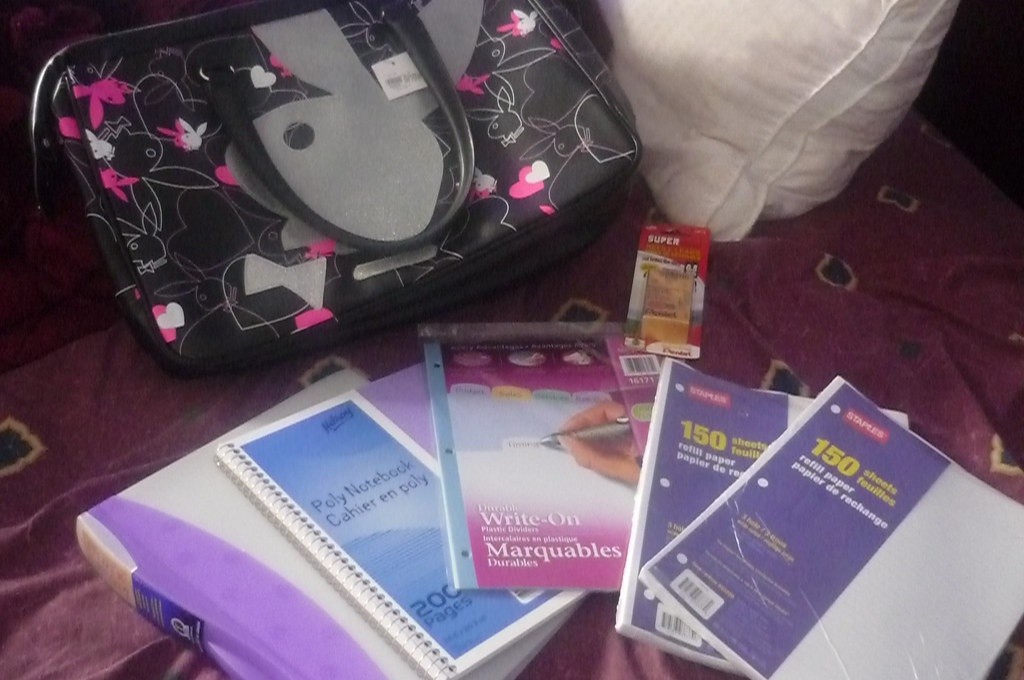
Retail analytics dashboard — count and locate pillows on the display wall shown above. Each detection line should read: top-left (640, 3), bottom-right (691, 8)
top-left (598, 0), bottom-right (960, 248)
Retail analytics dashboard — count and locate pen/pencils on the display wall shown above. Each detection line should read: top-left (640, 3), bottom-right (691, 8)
top-left (540, 415), bottom-right (631, 446)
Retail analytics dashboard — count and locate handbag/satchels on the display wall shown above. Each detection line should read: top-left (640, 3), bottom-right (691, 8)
top-left (28, 0), bottom-right (644, 379)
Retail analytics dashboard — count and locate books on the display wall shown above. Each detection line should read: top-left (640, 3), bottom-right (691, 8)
top-left (613, 359), bottom-right (1023, 680)
top-left (213, 362), bottom-right (595, 679)
top-left (77, 365), bottom-right (587, 679)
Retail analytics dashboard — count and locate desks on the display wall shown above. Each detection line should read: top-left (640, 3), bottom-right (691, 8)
top-left (0, 108), bottom-right (1024, 680)
